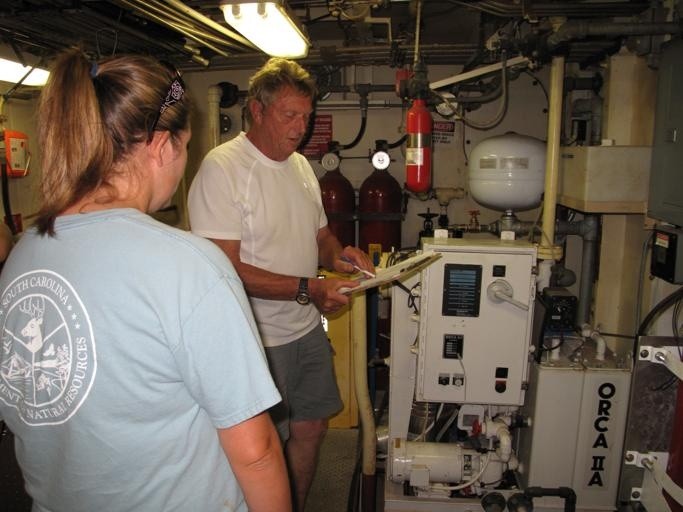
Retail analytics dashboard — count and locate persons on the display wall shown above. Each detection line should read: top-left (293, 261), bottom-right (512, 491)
top-left (0, 50), bottom-right (292, 512)
top-left (187, 58), bottom-right (375, 512)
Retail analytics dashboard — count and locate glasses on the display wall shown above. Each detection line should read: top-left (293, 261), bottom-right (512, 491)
top-left (149, 58), bottom-right (186, 145)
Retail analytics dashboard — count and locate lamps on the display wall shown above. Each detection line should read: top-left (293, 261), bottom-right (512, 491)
top-left (220, 0), bottom-right (312, 60)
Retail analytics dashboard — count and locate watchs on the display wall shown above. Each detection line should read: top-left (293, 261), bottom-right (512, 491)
top-left (295, 277), bottom-right (311, 306)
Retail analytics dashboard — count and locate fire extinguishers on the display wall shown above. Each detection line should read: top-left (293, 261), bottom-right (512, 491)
top-left (405, 99), bottom-right (432, 192)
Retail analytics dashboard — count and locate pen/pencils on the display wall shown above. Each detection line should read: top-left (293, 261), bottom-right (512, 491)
top-left (341, 256), bottom-right (377, 278)
